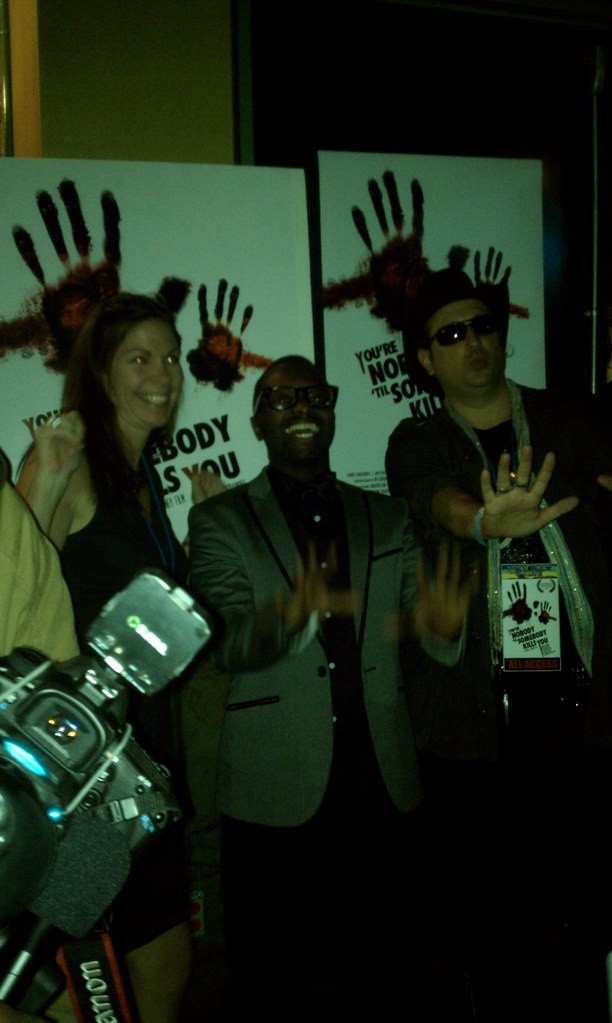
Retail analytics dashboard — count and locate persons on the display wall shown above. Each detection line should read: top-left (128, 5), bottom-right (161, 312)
top-left (190, 355), bottom-right (473, 1023)
top-left (385, 270), bottom-right (612, 1023)
top-left (16, 293), bottom-right (190, 1023)
top-left (0, 453), bottom-right (80, 664)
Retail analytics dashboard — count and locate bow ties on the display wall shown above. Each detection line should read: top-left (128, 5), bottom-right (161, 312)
top-left (287, 471), bottom-right (336, 509)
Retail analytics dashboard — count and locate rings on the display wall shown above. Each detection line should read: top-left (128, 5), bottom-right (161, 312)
top-left (48, 418), bottom-right (61, 427)
top-left (514, 481), bottom-right (529, 488)
top-left (499, 486), bottom-right (512, 494)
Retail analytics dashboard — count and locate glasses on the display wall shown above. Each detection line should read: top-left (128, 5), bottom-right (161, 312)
top-left (427, 313), bottom-right (501, 347)
top-left (254, 384), bottom-right (338, 417)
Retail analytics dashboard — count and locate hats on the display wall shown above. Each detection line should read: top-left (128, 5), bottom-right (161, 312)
top-left (402, 268), bottom-right (511, 396)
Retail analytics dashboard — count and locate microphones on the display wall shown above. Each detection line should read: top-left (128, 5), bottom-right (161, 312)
top-left (0, 816), bottom-right (133, 1011)
